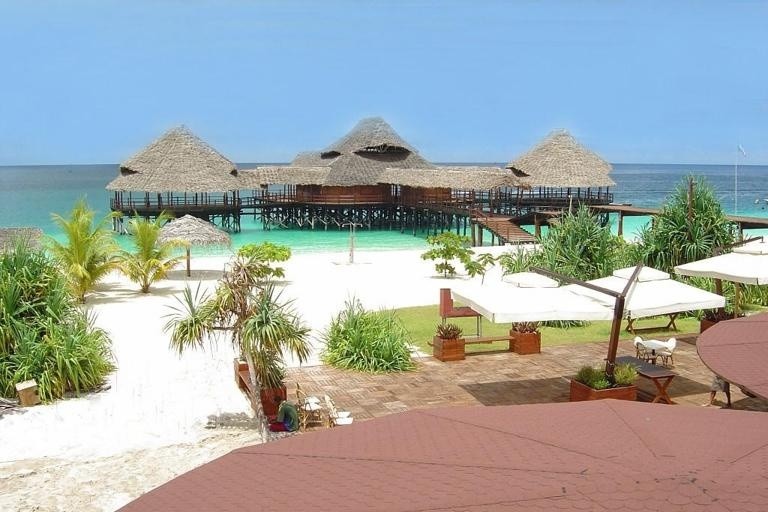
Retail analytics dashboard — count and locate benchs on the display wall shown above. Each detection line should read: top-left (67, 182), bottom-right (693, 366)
top-left (239, 370), bottom-right (253, 397)
top-left (604, 356), bottom-right (680, 404)
top-left (463, 335), bottom-right (514, 345)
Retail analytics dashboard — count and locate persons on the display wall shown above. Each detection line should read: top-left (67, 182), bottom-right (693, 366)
top-left (266, 395), bottom-right (299, 431)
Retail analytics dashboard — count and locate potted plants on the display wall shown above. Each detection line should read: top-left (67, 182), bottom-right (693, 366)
top-left (433, 322), bottom-right (465, 362)
top-left (569, 360), bottom-right (642, 402)
top-left (509, 321), bottom-right (541, 355)
top-left (253, 347), bottom-right (288, 416)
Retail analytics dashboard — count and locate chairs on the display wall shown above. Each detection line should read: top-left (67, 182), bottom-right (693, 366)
top-left (295, 382), bottom-right (338, 430)
